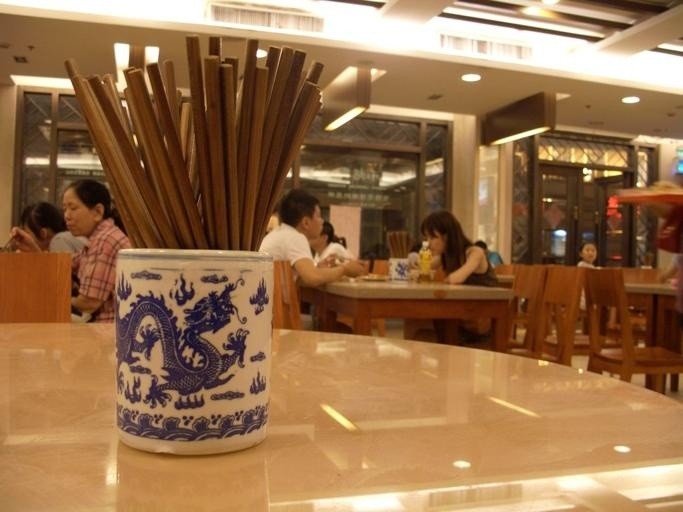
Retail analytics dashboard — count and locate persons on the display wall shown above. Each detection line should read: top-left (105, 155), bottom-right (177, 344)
top-left (257, 190), bottom-right (506, 347)
top-left (9, 180), bottom-right (133, 323)
top-left (576, 241), bottom-right (621, 331)
top-left (657, 253), bottom-right (683, 327)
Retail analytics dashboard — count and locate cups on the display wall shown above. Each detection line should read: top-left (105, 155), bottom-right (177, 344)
top-left (112, 246), bottom-right (274, 455)
top-left (388, 257), bottom-right (410, 281)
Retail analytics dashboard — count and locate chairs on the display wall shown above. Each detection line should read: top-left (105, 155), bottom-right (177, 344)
top-left (268, 253), bottom-right (586, 367)
top-left (587, 265), bottom-right (683, 393)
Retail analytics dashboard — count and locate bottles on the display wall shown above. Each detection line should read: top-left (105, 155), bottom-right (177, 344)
top-left (418, 240), bottom-right (433, 285)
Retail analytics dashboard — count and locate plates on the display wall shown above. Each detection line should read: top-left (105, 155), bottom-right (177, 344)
top-left (359, 275), bottom-right (386, 282)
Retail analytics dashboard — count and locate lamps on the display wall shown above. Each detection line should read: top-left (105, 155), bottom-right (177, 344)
top-left (321, 66), bottom-right (370, 133)
top-left (480, 91), bottom-right (557, 149)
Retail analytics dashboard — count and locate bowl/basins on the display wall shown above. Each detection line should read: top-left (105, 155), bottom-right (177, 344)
top-left (409, 268), bottom-right (435, 282)
